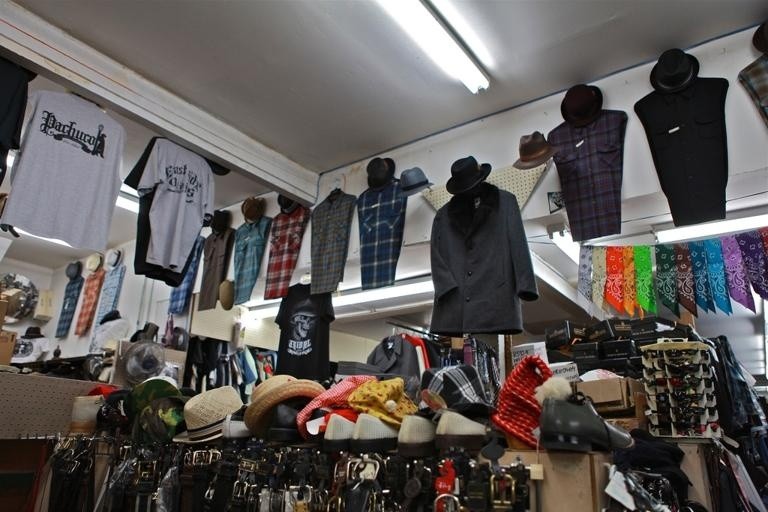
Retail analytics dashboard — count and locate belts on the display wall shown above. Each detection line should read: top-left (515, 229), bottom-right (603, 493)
top-left (24, 438), bottom-right (530, 512)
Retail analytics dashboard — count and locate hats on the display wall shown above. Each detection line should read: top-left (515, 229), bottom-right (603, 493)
top-left (66, 248), bottom-right (123, 280)
top-left (512, 132), bottom-right (563, 170)
top-left (69, 339), bottom-right (491, 444)
top-left (752, 20), bottom-right (768, 53)
top-left (20, 326), bottom-right (45, 339)
top-left (366, 157), bottom-right (435, 198)
top-left (492, 354), bottom-right (552, 449)
top-left (208, 194), bottom-right (302, 233)
top-left (650, 48), bottom-right (700, 94)
top-left (561, 83), bottom-right (604, 127)
top-left (446, 156), bottom-right (492, 195)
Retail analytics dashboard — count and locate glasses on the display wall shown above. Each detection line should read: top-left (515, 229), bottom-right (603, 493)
top-left (639, 346), bottom-right (726, 439)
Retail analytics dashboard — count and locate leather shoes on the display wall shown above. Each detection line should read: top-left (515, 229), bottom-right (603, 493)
top-left (540, 392), bottom-right (635, 453)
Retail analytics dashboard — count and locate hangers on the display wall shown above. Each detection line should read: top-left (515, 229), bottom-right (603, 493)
top-left (369, 318), bottom-right (446, 365)
